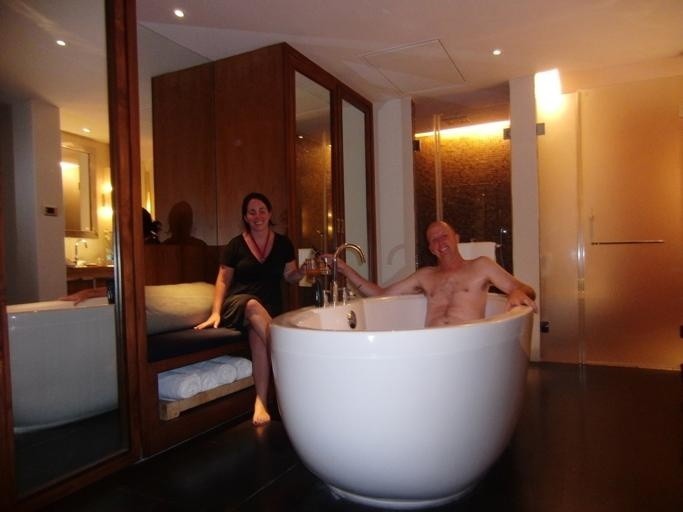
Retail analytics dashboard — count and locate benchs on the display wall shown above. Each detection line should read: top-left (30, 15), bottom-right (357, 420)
top-left (145, 324), bottom-right (250, 360)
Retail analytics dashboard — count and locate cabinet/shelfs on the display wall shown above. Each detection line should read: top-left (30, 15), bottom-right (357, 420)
top-left (220, 41), bottom-right (378, 303)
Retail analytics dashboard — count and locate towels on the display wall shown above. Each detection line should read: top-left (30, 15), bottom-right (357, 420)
top-left (156, 352), bottom-right (253, 402)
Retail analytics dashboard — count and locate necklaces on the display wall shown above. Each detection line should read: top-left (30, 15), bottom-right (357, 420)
top-left (248, 227), bottom-right (271, 262)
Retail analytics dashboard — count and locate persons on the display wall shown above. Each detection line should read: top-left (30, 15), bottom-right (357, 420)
top-left (58, 285), bottom-right (106, 306)
top-left (191, 191), bottom-right (319, 427)
top-left (318, 220), bottom-right (539, 330)
top-left (139, 208), bottom-right (164, 245)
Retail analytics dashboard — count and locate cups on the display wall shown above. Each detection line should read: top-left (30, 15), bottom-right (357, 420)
top-left (76, 260), bottom-right (87, 266)
top-left (305, 257), bottom-right (320, 277)
top-left (318, 256), bottom-right (332, 276)
top-left (95, 256), bottom-right (106, 267)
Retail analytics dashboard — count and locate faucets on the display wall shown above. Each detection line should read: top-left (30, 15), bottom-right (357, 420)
top-left (74, 240), bottom-right (88, 263)
top-left (332, 242), bottom-right (366, 303)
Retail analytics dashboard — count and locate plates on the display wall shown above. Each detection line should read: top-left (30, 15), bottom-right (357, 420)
top-left (74, 266), bottom-right (88, 268)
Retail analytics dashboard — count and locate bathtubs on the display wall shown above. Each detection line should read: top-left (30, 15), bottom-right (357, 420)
top-left (269, 292), bottom-right (533, 509)
top-left (6, 299), bottom-right (118, 435)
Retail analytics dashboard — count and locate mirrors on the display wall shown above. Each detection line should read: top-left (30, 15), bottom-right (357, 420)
top-left (60, 141), bottom-right (100, 240)
top-left (284, 44), bottom-right (377, 301)
top-left (0, 2), bottom-right (137, 510)
top-left (136, 21), bottom-right (217, 249)
